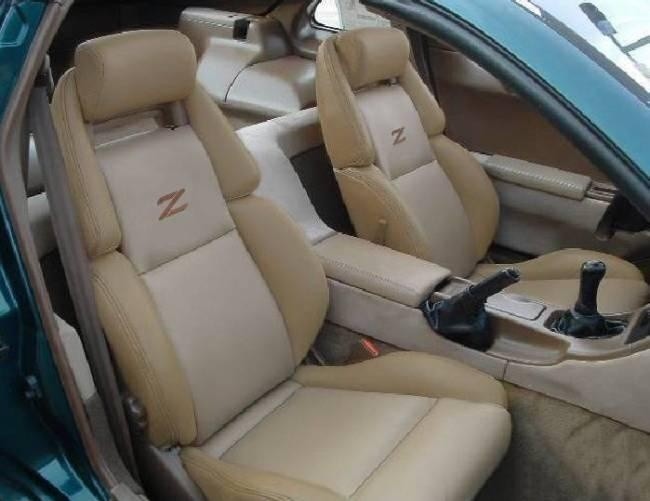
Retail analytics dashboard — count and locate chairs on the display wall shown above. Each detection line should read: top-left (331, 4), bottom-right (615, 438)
top-left (48, 23), bottom-right (517, 501)
top-left (312, 25), bottom-right (650, 324)
